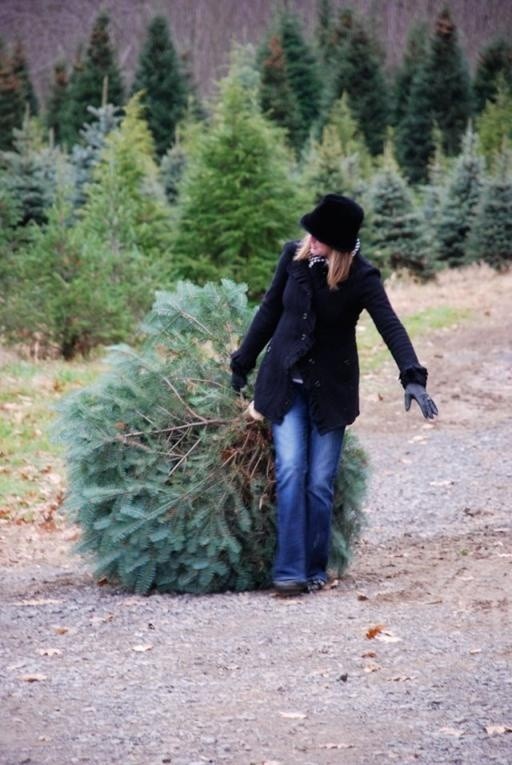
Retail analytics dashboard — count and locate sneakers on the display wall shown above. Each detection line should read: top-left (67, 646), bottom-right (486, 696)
top-left (273, 579), bottom-right (326, 595)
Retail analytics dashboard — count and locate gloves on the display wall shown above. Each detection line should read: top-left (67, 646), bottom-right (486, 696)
top-left (404, 383), bottom-right (438, 419)
top-left (232, 372), bottom-right (247, 392)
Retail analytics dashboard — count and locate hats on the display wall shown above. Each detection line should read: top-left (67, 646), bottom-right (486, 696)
top-left (301, 194), bottom-right (365, 252)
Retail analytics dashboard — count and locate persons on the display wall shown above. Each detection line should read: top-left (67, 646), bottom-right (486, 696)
top-left (230, 194), bottom-right (439, 594)
